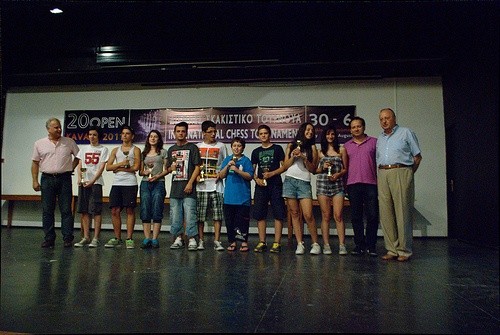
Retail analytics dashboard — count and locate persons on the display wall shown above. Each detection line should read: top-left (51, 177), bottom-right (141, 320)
top-left (138, 129), bottom-right (171, 248)
top-left (195, 108), bottom-right (422, 260)
top-left (30, 118), bottom-right (78, 248)
top-left (166, 122), bottom-right (200, 249)
top-left (74, 126), bottom-right (109, 247)
top-left (105, 125), bottom-right (140, 248)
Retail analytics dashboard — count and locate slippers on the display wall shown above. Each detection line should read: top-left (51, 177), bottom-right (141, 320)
top-left (227, 242), bottom-right (237, 251)
top-left (240, 244), bottom-right (249, 252)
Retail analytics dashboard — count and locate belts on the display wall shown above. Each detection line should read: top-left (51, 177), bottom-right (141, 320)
top-left (42, 172), bottom-right (61, 177)
top-left (379, 164), bottom-right (412, 169)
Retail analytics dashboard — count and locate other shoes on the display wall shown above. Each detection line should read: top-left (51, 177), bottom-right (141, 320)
top-left (323, 244), bottom-right (332, 254)
top-left (64, 239), bottom-right (72, 247)
top-left (41, 240), bottom-right (54, 247)
top-left (367, 249), bottom-right (378, 256)
top-left (351, 248), bottom-right (364, 255)
top-left (338, 245), bottom-right (348, 254)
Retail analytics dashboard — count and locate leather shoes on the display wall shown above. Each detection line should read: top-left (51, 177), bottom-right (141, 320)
top-left (397, 256), bottom-right (410, 261)
top-left (381, 254), bottom-right (398, 260)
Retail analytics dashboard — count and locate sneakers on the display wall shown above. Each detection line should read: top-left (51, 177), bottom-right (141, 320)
top-left (170, 236), bottom-right (186, 249)
top-left (88, 238), bottom-right (102, 247)
top-left (104, 237), bottom-right (123, 247)
top-left (213, 240), bottom-right (224, 250)
top-left (125, 238), bottom-right (135, 249)
top-left (310, 243), bottom-right (321, 254)
top-left (188, 237), bottom-right (198, 250)
top-left (270, 242), bottom-right (282, 253)
top-left (254, 242), bottom-right (268, 252)
top-left (151, 239), bottom-right (159, 248)
top-left (295, 241), bottom-right (305, 254)
top-left (140, 238), bottom-right (152, 248)
top-left (73, 237), bottom-right (92, 247)
top-left (196, 239), bottom-right (205, 250)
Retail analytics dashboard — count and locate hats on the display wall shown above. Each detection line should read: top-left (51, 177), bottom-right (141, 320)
top-left (176, 122), bottom-right (188, 130)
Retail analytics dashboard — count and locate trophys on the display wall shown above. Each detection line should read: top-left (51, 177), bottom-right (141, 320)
top-left (326, 163), bottom-right (333, 178)
top-left (79, 167), bottom-right (87, 184)
top-left (171, 156), bottom-right (177, 175)
top-left (122, 147), bottom-right (131, 169)
top-left (229, 155), bottom-right (238, 173)
top-left (145, 159), bottom-right (155, 179)
top-left (198, 164), bottom-right (206, 181)
top-left (295, 140), bottom-right (302, 157)
top-left (262, 167), bottom-right (269, 186)
